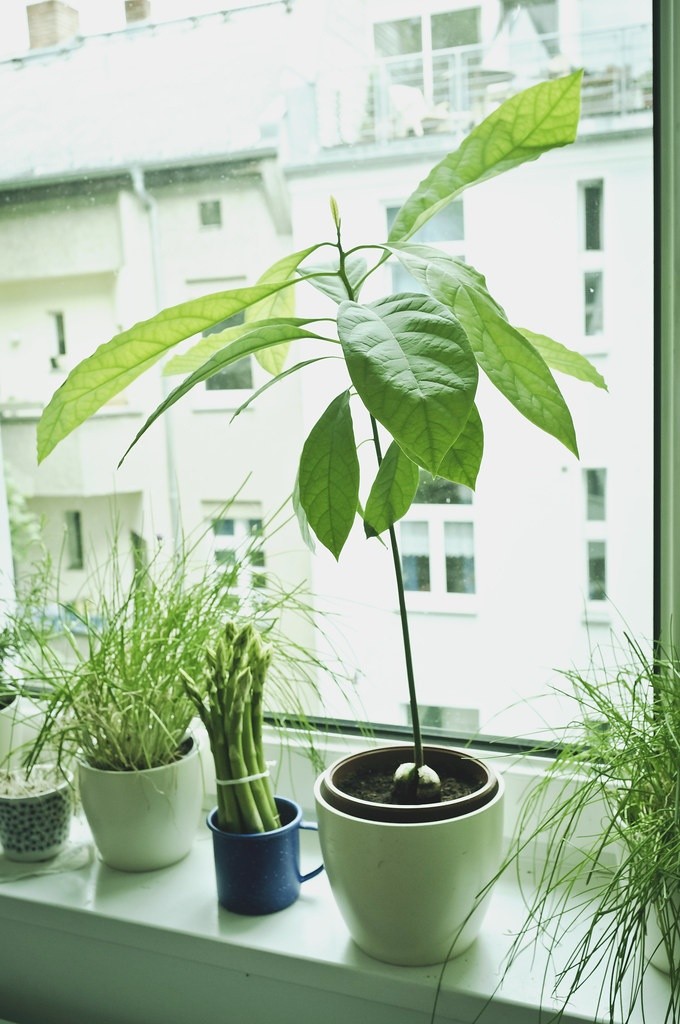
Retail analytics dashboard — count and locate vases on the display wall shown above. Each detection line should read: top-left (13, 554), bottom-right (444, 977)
top-left (0, 762), bottom-right (75, 862)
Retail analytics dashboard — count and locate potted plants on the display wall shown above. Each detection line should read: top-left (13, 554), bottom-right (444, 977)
top-left (34, 68), bottom-right (610, 967)
top-left (14, 471), bottom-right (206, 874)
top-left (0, 629), bottom-right (20, 766)
top-left (174, 623), bottom-right (328, 917)
top-left (507, 634), bottom-right (680, 977)
